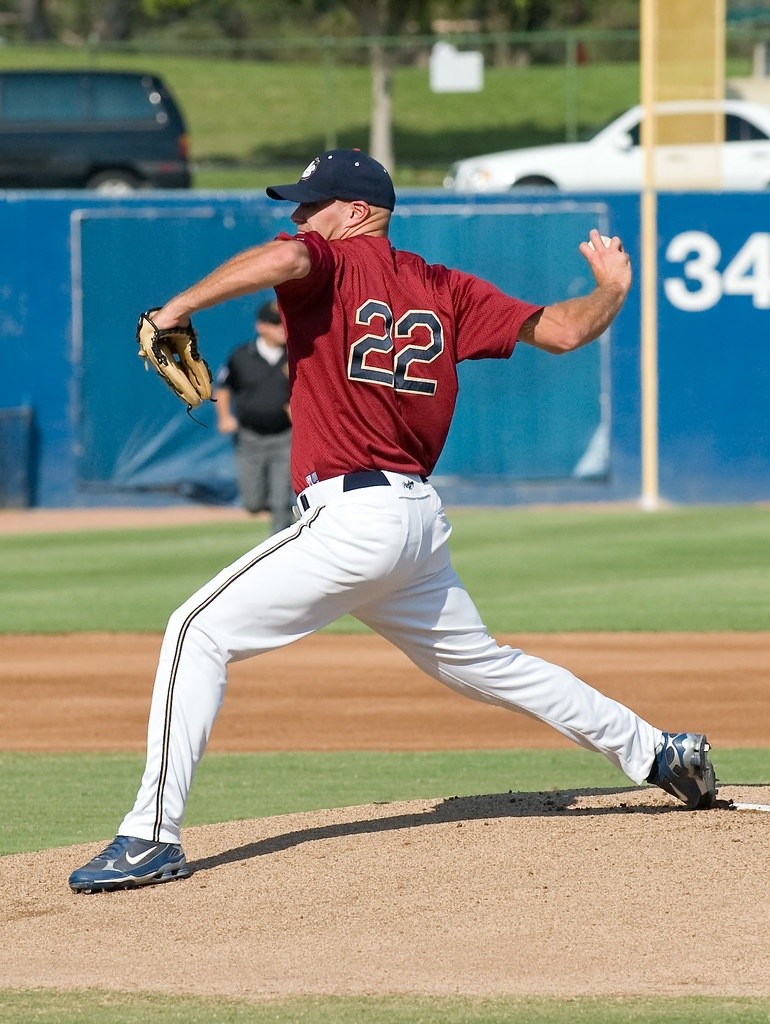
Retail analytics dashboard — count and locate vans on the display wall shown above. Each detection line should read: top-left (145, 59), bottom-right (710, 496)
top-left (0, 71), bottom-right (193, 189)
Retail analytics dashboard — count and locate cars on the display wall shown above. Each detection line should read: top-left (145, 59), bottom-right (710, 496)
top-left (443, 98), bottom-right (770, 191)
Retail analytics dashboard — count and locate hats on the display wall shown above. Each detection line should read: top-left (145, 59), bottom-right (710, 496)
top-left (266, 148), bottom-right (395, 212)
top-left (256, 301), bottom-right (282, 325)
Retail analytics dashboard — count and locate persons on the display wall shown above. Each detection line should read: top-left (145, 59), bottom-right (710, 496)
top-left (215, 300), bottom-right (293, 534)
top-left (70, 148), bottom-right (717, 894)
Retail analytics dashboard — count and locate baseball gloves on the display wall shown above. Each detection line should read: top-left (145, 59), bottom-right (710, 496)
top-left (136, 304), bottom-right (222, 426)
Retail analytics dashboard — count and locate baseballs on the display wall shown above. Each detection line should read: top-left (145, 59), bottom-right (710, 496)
top-left (588, 234), bottom-right (615, 253)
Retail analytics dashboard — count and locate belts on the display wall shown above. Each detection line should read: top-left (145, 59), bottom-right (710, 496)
top-left (300, 470), bottom-right (428, 511)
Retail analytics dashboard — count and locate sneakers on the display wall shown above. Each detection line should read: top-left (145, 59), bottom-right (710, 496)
top-left (69, 836), bottom-right (189, 893)
top-left (646, 733), bottom-right (719, 811)
